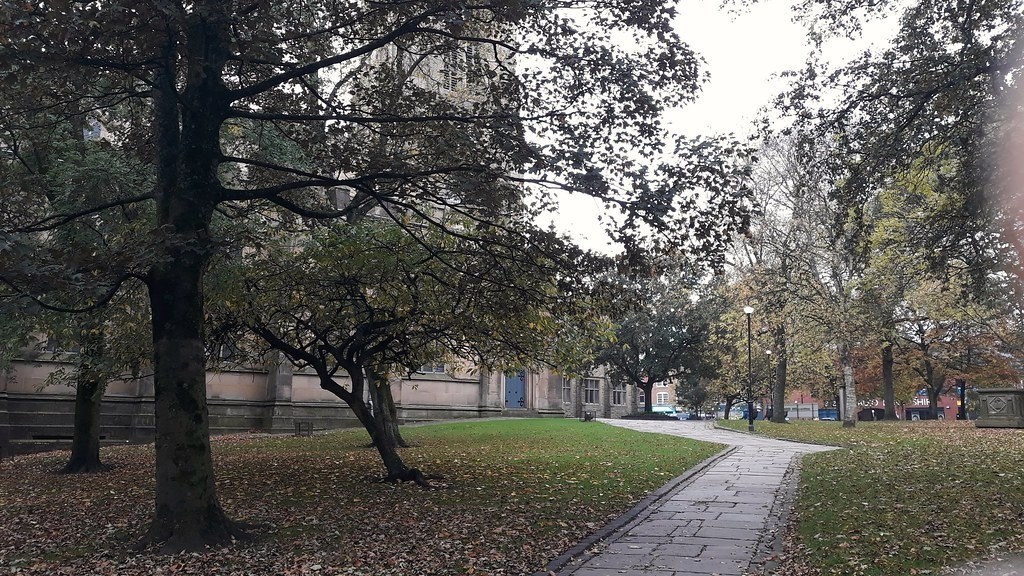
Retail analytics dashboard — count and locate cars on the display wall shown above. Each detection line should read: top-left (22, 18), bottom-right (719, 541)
top-left (690, 408), bottom-right (714, 419)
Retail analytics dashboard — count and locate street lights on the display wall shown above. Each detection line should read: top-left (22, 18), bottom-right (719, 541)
top-left (794, 401), bottom-right (799, 418)
top-left (744, 306), bottom-right (754, 431)
top-left (766, 350), bottom-right (773, 419)
